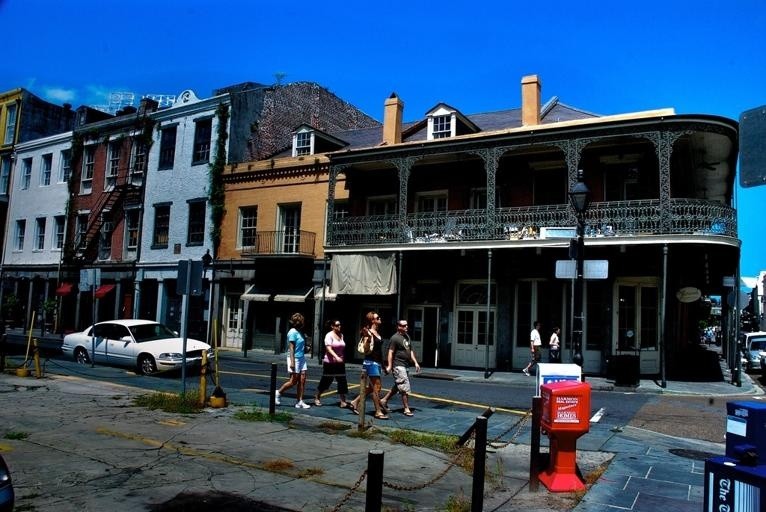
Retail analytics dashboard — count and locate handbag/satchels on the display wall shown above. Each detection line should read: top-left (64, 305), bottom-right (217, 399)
top-left (305, 336), bottom-right (310, 354)
top-left (357, 335), bottom-right (374, 354)
top-left (549, 350), bottom-right (559, 362)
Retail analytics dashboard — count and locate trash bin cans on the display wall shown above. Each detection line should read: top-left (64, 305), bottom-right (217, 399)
top-left (615, 346), bottom-right (641, 387)
top-left (716, 331), bottom-right (721, 346)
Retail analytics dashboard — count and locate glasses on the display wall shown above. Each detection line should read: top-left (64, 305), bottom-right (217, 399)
top-left (373, 317), bottom-right (379, 320)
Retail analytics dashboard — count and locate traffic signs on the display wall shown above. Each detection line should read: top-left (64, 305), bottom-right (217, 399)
top-left (568, 168), bottom-right (592, 386)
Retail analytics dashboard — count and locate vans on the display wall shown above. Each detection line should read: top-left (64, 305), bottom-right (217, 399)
top-left (744, 332), bottom-right (766, 358)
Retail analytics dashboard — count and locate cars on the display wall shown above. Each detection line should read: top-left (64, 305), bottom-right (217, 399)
top-left (748, 338), bottom-right (766, 370)
top-left (62, 319), bottom-right (214, 375)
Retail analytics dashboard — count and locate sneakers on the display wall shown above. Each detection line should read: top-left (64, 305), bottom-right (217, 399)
top-left (380, 399), bottom-right (392, 412)
top-left (295, 400), bottom-right (311, 409)
top-left (523, 369), bottom-right (531, 376)
top-left (275, 390), bottom-right (281, 405)
top-left (404, 408), bottom-right (414, 416)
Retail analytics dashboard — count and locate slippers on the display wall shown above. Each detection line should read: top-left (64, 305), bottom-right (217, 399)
top-left (315, 393), bottom-right (322, 406)
top-left (340, 404), bottom-right (354, 408)
top-left (350, 408), bottom-right (359, 415)
top-left (375, 415), bottom-right (389, 420)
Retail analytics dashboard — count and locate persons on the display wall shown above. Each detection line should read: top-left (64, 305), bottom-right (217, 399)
top-left (549, 326), bottom-right (561, 362)
top-left (275, 313), bottom-right (311, 409)
top-left (350, 311), bottom-right (390, 420)
top-left (523, 320), bottom-right (542, 377)
top-left (313, 319), bottom-right (350, 409)
top-left (379, 319), bottom-right (421, 417)
top-left (706, 327), bottom-right (713, 348)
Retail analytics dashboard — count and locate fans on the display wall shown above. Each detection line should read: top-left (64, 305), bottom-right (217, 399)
top-left (697, 150), bottom-right (720, 170)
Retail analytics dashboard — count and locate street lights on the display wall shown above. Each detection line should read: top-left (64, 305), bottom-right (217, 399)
top-left (198, 249), bottom-right (212, 333)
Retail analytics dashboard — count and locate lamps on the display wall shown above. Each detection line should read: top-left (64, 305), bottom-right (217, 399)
top-left (536, 248), bottom-right (541, 255)
top-left (620, 245), bottom-right (626, 253)
top-left (461, 250), bottom-right (465, 256)
